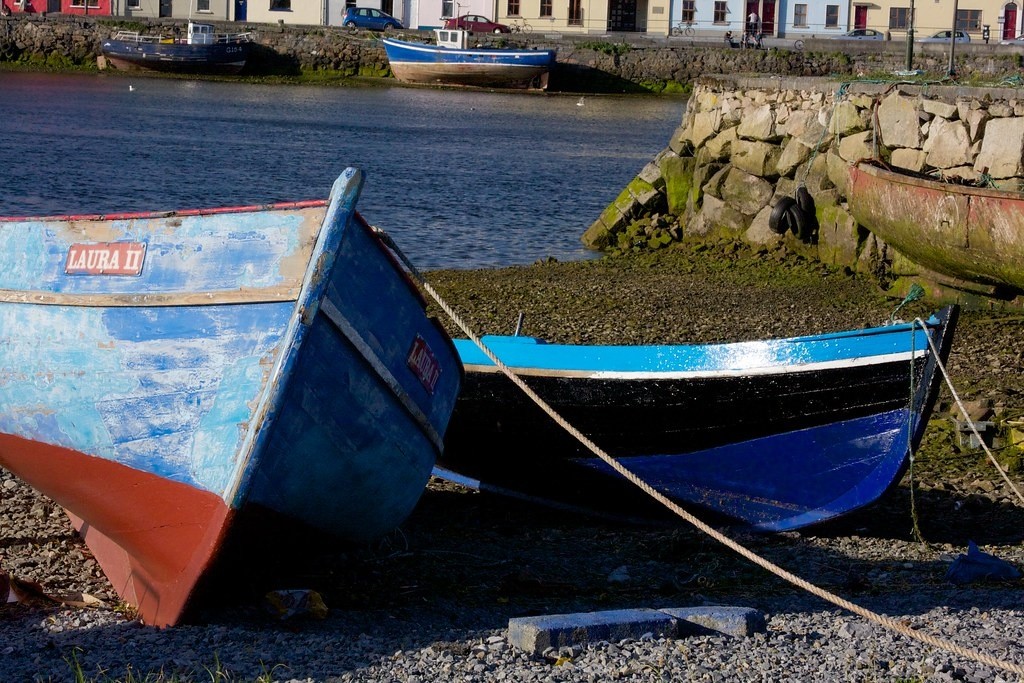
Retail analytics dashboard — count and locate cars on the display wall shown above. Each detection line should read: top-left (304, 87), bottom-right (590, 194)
top-left (999, 33), bottom-right (1024, 47)
top-left (342, 7), bottom-right (405, 32)
top-left (914, 29), bottom-right (971, 42)
top-left (829, 28), bottom-right (893, 39)
top-left (444, 14), bottom-right (512, 35)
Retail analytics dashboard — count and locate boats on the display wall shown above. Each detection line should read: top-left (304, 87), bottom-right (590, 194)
top-left (845, 160), bottom-right (1024, 299)
top-left (380, 30), bottom-right (557, 91)
top-left (429, 293), bottom-right (965, 539)
top-left (1, 163), bottom-right (466, 631)
top-left (100, 21), bottom-right (257, 74)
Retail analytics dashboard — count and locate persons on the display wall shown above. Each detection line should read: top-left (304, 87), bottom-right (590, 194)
top-left (724, 30), bottom-right (764, 50)
top-left (748, 10), bottom-right (758, 36)
top-left (2, 0), bottom-right (28, 17)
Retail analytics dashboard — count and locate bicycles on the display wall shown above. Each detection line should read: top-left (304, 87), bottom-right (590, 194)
top-left (793, 34), bottom-right (816, 50)
top-left (671, 21), bottom-right (696, 37)
top-left (509, 16), bottom-right (533, 36)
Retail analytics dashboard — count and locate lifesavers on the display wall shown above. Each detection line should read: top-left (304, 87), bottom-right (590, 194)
top-left (787, 205), bottom-right (806, 240)
top-left (795, 187), bottom-right (812, 228)
top-left (769, 186), bottom-right (797, 235)
top-left (1, 42), bottom-right (17, 59)
